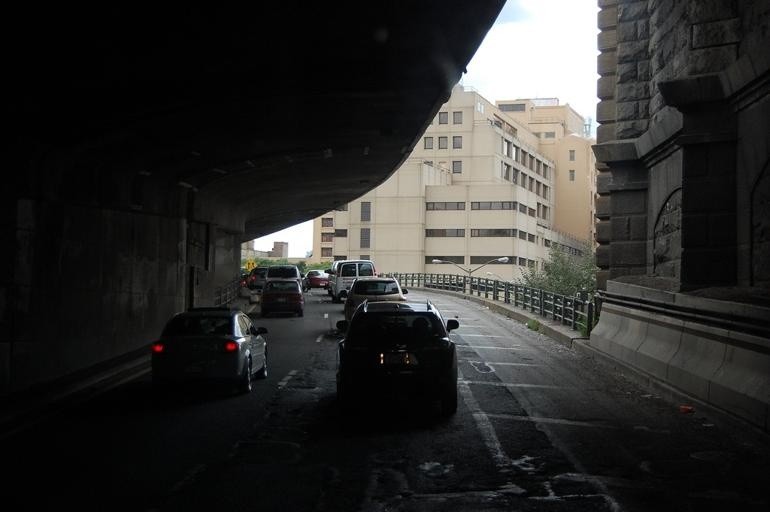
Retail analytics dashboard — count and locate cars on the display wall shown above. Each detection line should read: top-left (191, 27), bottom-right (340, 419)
top-left (338, 274), bottom-right (408, 321)
top-left (242, 264), bottom-right (304, 317)
top-left (151, 307), bottom-right (268, 392)
top-left (336, 299), bottom-right (458, 412)
top-left (306, 270), bottom-right (330, 288)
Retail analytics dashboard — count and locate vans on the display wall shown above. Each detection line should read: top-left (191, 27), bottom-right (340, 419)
top-left (325, 259), bottom-right (375, 301)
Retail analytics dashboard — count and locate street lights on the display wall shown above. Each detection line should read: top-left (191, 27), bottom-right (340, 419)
top-left (432, 256), bottom-right (510, 279)
